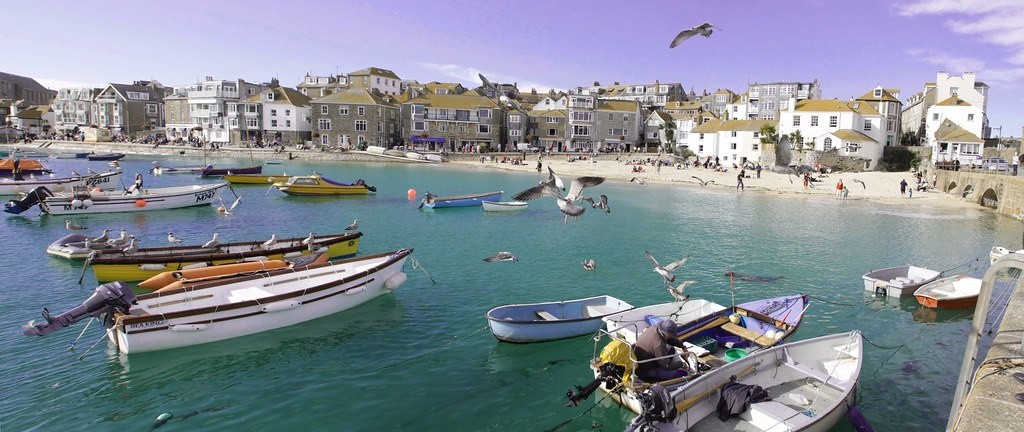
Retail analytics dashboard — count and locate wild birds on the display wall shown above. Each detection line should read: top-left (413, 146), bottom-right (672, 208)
top-left (670, 22), bottom-right (722, 50)
top-left (92, 227), bottom-right (141, 258)
top-left (218, 196), bottom-right (241, 215)
top-left (581, 260), bottom-right (595, 271)
top-left (483, 251), bottom-right (518, 263)
top-left (64, 220), bottom-right (88, 233)
top-left (692, 175), bottom-right (715, 187)
top-left (478, 74), bottom-right (529, 118)
top-left (263, 234), bottom-right (277, 248)
top-left (167, 232), bottom-right (183, 246)
top-left (644, 250), bottom-right (690, 285)
top-left (202, 233), bottom-right (220, 249)
top-left (302, 232), bottom-right (317, 244)
top-left (511, 165), bottom-right (611, 224)
top-left (344, 219), bottom-right (360, 232)
top-left (630, 177), bottom-right (647, 186)
top-left (666, 279), bottom-right (702, 302)
top-left (850, 178), bottom-right (866, 190)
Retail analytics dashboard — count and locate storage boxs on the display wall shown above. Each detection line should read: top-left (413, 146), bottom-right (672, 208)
top-left (721, 336), bottom-right (750, 348)
top-left (690, 336), bottom-right (719, 354)
top-left (716, 330), bottom-right (740, 344)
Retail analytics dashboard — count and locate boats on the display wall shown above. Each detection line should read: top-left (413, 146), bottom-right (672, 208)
top-left (273, 173), bottom-right (377, 194)
top-left (52, 151), bottom-right (126, 161)
top-left (34, 182), bottom-right (229, 216)
top-left (418, 190), bottom-right (503, 209)
top-left (485, 294), bottom-right (634, 343)
top-left (913, 275), bottom-right (983, 308)
top-left (21, 248), bottom-right (415, 353)
top-left (624, 331), bottom-right (864, 432)
top-left (565, 281), bottom-right (804, 418)
top-left (202, 116), bottom-right (262, 175)
top-left (86, 231), bottom-right (363, 283)
top-left (48, 232), bottom-right (126, 261)
top-left (0, 167), bottom-right (123, 196)
top-left (4, 186), bottom-right (50, 214)
top-left (0, 123), bottom-right (52, 175)
top-left (482, 200), bottom-right (529, 212)
top-left (148, 160), bottom-right (207, 174)
top-left (862, 265), bottom-right (941, 299)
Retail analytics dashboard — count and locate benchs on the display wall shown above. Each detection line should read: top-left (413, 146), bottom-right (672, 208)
top-left (928, 288), bottom-right (960, 297)
top-left (536, 310), bottom-right (558, 320)
top-left (720, 322), bottom-right (774, 347)
top-left (725, 389), bottom-right (812, 432)
top-left (778, 359), bottom-right (846, 390)
top-left (680, 342), bottom-right (725, 369)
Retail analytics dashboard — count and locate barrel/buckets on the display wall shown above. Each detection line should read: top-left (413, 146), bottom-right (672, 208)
top-left (724, 348), bottom-right (749, 364)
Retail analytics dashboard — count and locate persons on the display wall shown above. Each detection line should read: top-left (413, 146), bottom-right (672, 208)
top-left (633, 319), bottom-right (689, 383)
top-left (437, 142), bottom-right (761, 190)
top-left (250, 136), bottom-right (353, 152)
top-left (1013, 152), bottom-right (1024, 176)
top-left (900, 157), bottom-right (960, 200)
top-left (110, 134), bottom-right (220, 149)
top-left (803, 162), bottom-right (850, 199)
top-left (36, 130), bottom-right (86, 142)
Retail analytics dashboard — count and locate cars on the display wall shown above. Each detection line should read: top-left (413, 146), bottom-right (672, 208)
top-left (982, 157), bottom-right (1009, 171)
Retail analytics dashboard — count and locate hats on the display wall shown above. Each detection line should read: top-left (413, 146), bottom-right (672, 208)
top-left (658, 319), bottom-right (678, 337)
